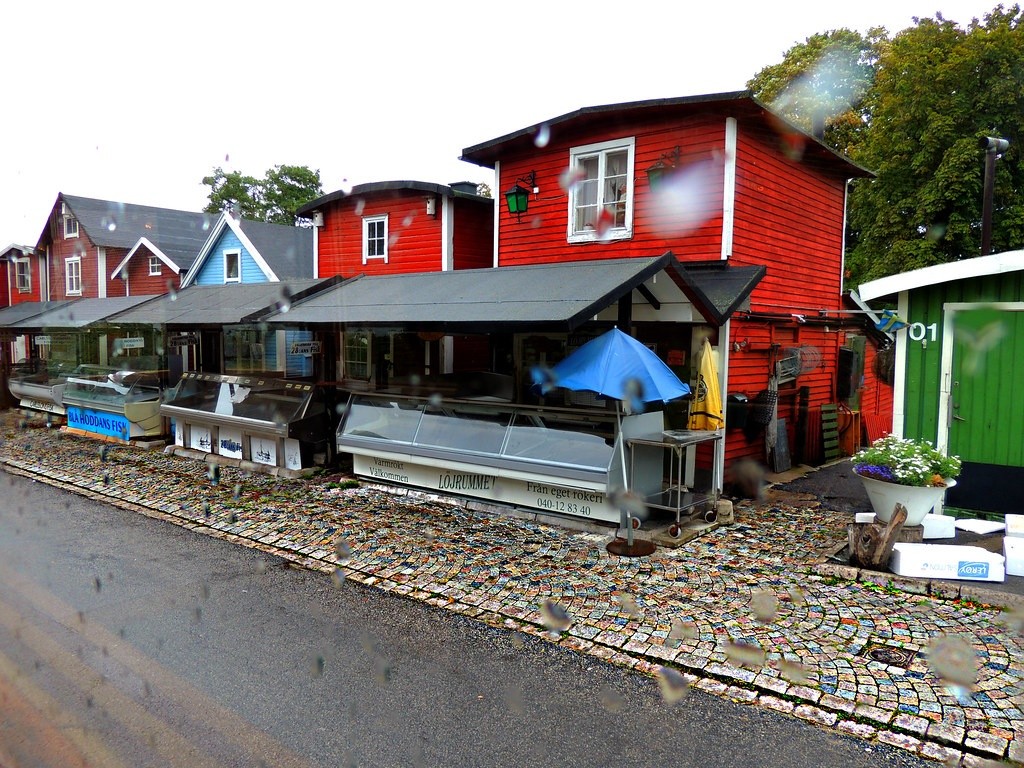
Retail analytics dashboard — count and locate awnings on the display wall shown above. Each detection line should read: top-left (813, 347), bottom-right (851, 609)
top-left (0, 298), bottom-right (80, 325)
top-left (270, 250), bottom-right (723, 326)
top-left (0, 294), bottom-right (162, 333)
top-left (107, 275), bottom-right (341, 325)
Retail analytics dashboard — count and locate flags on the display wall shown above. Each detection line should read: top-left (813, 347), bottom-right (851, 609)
top-left (875, 310), bottom-right (911, 334)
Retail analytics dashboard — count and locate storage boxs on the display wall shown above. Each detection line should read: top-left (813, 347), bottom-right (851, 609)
top-left (1002, 536), bottom-right (1024, 577)
top-left (856, 507), bottom-right (956, 539)
top-left (890, 542), bottom-right (1005, 582)
top-left (1005, 514), bottom-right (1024, 537)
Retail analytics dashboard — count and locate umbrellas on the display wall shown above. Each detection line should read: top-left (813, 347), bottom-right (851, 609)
top-left (530, 324), bottom-right (692, 516)
top-left (686, 336), bottom-right (726, 431)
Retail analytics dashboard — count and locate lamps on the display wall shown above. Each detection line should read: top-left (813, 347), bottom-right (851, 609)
top-left (645, 146), bottom-right (682, 195)
top-left (503, 170), bottom-right (541, 223)
top-left (426, 197), bottom-right (436, 219)
top-left (313, 211), bottom-right (326, 232)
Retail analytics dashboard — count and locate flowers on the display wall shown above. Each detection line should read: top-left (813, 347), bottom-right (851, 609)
top-left (852, 430), bottom-right (962, 486)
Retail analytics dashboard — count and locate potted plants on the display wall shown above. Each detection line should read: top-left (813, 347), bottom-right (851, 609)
top-left (852, 467), bottom-right (958, 526)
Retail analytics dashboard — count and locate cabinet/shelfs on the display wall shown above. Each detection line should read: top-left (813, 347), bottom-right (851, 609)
top-left (627, 429), bottom-right (723, 524)
top-left (838, 411), bottom-right (860, 456)
